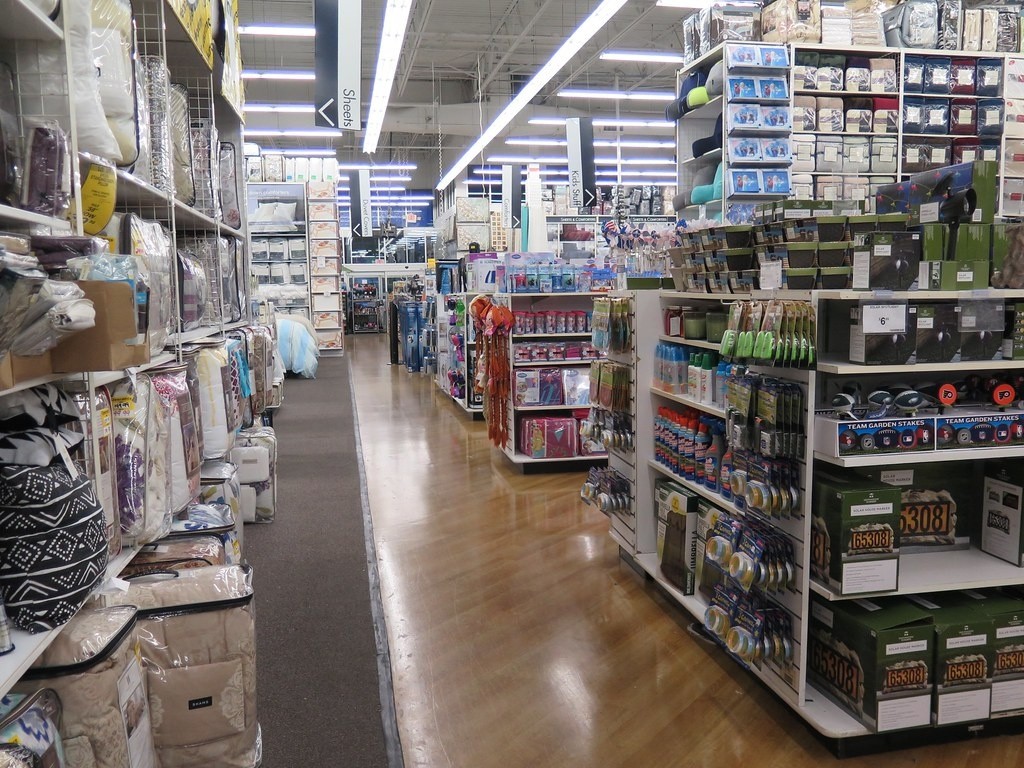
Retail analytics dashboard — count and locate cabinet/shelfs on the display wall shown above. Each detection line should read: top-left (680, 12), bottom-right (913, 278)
top-left (0, 1), bottom-right (256, 767)
top-left (430, 40), bottom-right (1023, 768)
top-left (246, 181), bottom-right (312, 325)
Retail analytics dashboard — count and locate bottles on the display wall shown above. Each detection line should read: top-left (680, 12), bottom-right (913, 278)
top-left (653, 345), bottom-right (732, 410)
top-left (513, 311), bottom-right (593, 333)
top-left (664, 304), bottom-right (730, 343)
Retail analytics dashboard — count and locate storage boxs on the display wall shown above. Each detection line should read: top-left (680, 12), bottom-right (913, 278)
top-left (808, 463), bottom-right (901, 596)
top-left (981, 464), bottom-right (1023, 568)
top-left (907, 159), bottom-right (996, 226)
top-left (912, 593), bottom-right (992, 727)
top-left (806, 588), bottom-right (935, 734)
top-left (961, 588), bottom-right (1024, 721)
top-left (875, 180), bottom-right (912, 213)
